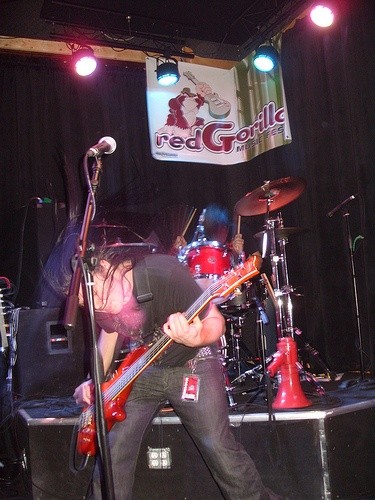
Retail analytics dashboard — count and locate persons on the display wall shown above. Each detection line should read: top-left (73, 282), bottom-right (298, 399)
top-left (174, 204), bottom-right (314, 382)
top-left (41, 226), bottom-right (283, 498)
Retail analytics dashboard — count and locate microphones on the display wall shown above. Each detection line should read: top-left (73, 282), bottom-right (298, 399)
top-left (85, 136), bottom-right (116, 157)
top-left (328, 195), bottom-right (356, 217)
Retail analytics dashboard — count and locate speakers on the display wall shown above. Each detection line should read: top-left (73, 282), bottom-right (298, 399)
top-left (16, 201), bottom-right (66, 309)
top-left (10, 308), bottom-right (91, 401)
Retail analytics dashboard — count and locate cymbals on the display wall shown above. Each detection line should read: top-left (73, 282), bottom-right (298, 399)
top-left (253, 227), bottom-right (301, 239)
top-left (234, 175), bottom-right (307, 216)
top-left (91, 223), bottom-right (128, 229)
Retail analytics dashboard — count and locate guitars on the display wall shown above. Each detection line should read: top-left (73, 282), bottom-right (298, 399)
top-left (182, 70), bottom-right (231, 119)
top-left (76, 254), bottom-right (260, 458)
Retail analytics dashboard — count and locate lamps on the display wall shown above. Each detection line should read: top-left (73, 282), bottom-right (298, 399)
top-left (309, 0), bottom-right (335, 27)
top-left (155, 56), bottom-right (179, 85)
top-left (254, 46), bottom-right (278, 72)
top-left (73, 48), bottom-right (97, 76)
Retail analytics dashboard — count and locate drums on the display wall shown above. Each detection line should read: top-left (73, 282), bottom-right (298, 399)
top-left (219, 280), bottom-right (258, 317)
top-left (178, 241), bottom-right (236, 292)
top-left (94, 243), bottom-right (158, 255)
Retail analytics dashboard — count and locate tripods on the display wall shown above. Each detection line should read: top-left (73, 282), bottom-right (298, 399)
top-left (225, 208), bottom-right (337, 412)
